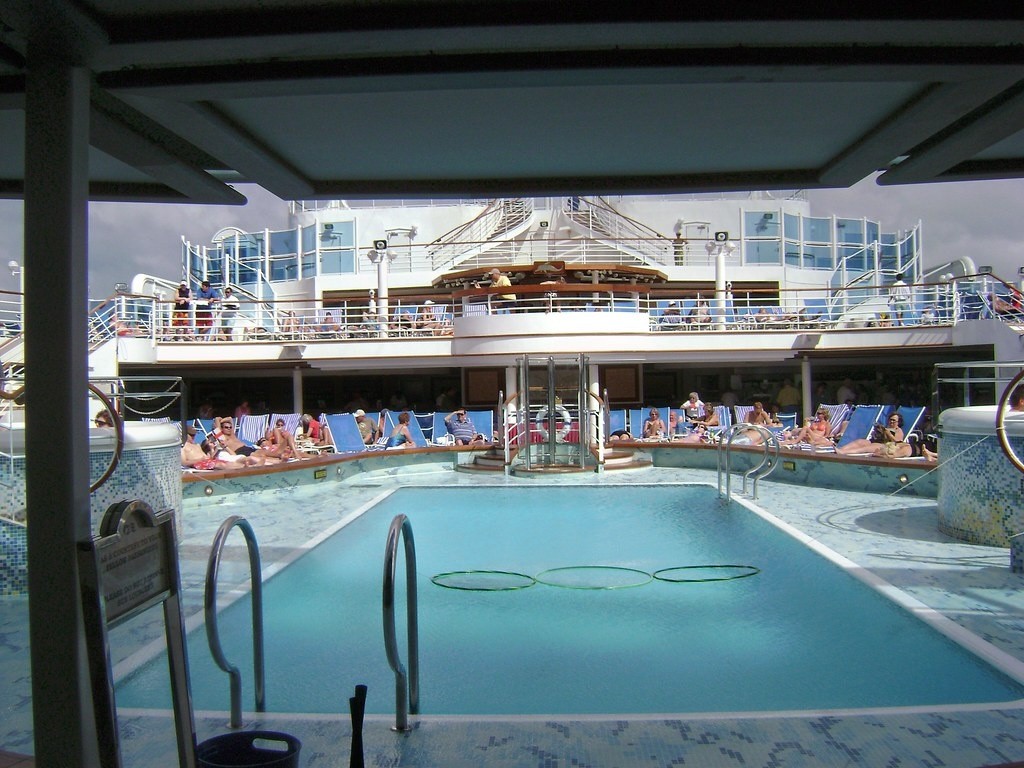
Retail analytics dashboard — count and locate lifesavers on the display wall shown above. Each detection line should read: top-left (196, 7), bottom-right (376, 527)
top-left (536, 407), bottom-right (572, 440)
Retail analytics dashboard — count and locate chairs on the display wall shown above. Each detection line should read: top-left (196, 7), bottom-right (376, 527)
top-left (0, 290), bottom-right (1023, 343)
top-left (602, 403), bottom-right (938, 462)
top-left (142, 411), bottom-right (495, 474)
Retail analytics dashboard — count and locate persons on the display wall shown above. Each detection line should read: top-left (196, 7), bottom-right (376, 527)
top-left (609, 374), bottom-right (981, 462)
top-left (921, 307), bottom-right (934, 324)
top-left (540, 277), bottom-right (566, 314)
top-left (475, 269), bottom-right (516, 314)
top-left (109, 280), bottom-right (240, 341)
top-left (196, 401), bottom-right (216, 425)
top-left (181, 413), bottom-right (334, 470)
top-left (980, 283), bottom-right (1024, 320)
top-left (1008, 384), bottom-right (1024, 412)
top-left (672, 232), bottom-right (689, 266)
top-left (867, 273), bottom-right (911, 327)
top-left (345, 385), bottom-right (489, 450)
top-left (662, 302), bottom-right (685, 323)
top-left (95, 411), bottom-right (116, 428)
top-left (687, 301), bottom-right (712, 327)
top-left (741, 309), bottom-right (822, 326)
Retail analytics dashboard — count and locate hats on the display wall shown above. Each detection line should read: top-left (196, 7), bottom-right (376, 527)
top-left (424, 300), bottom-right (435, 305)
top-left (352, 409), bottom-right (366, 418)
top-left (187, 425), bottom-right (199, 436)
top-left (181, 281), bottom-right (187, 286)
top-left (488, 268), bottom-right (501, 274)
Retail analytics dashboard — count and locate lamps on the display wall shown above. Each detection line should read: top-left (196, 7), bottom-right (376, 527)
top-left (8, 260), bottom-right (19, 276)
top-left (540, 221), bottom-right (549, 228)
top-left (366, 240), bottom-right (398, 263)
top-left (114, 282), bottom-right (127, 293)
top-left (938, 273), bottom-right (954, 284)
top-left (153, 288), bottom-right (166, 301)
top-left (706, 231), bottom-right (736, 256)
top-left (325, 223), bottom-right (334, 231)
top-left (978, 265), bottom-right (992, 273)
top-left (1017, 266), bottom-right (1024, 275)
top-left (762, 213), bottom-right (773, 220)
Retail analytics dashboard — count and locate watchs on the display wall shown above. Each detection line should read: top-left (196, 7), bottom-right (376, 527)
top-left (247, 299), bottom-right (454, 339)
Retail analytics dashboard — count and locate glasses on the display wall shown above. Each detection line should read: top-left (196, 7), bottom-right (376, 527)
top-left (95, 419), bottom-right (107, 426)
top-left (277, 424), bottom-right (284, 426)
top-left (222, 426), bottom-right (232, 429)
top-left (456, 414), bottom-right (464, 416)
top-left (817, 412), bottom-right (824, 415)
top-left (888, 419), bottom-right (899, 423)
top-left (651, 413), bottom-right (657, 415)
top-left (225, 291), bottom-right (230, 293)
top-left (260, 439), bottom-right (267, 444)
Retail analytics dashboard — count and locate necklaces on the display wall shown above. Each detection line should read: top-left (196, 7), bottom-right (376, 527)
top-left (235, 398), bottom-right (251, 435)
